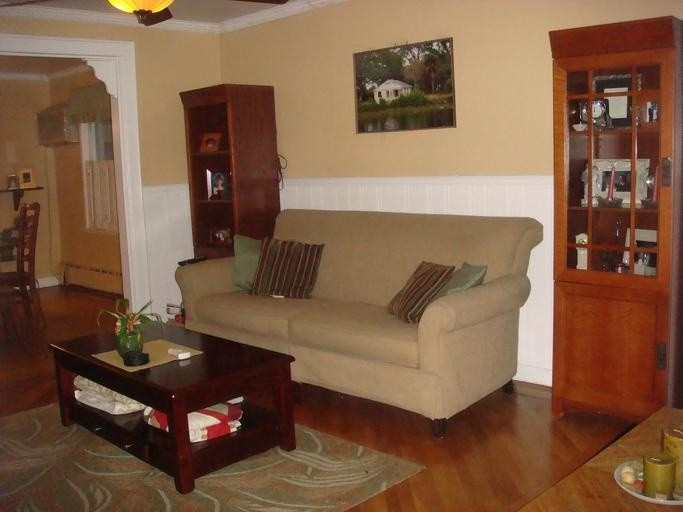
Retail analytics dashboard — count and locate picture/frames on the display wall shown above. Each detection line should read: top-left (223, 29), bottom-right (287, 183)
top-left (623, 227), bottom-right (657, 275)
top-left (584, 157), bottom-right (650, 205)
top-left (592, 73), bottom-right (641, 130)
top-left (205, 167), bottom-right (227, 199)
top-left (352, 37), bottom-right (456, 134)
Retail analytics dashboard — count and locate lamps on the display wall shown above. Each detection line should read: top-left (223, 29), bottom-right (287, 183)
top-left (108, 0), bottom-right (175, 24)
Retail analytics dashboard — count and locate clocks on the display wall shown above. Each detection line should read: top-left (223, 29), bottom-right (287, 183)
top-left (577, 99), bottom-right (614, 130)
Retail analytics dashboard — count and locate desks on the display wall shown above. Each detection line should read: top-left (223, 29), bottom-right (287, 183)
top-left (518, 406), bottom-right (683, 512)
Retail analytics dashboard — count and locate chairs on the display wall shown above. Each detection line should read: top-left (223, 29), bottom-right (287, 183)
top-left (0, 202), bottom-right (43, 320)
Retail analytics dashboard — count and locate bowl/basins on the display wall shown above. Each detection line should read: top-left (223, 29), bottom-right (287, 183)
top-left (573, 123), bottom-right (588, 132)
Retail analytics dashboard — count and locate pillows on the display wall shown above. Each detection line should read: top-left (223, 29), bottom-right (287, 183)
top-left (249, 237), bottom-right (325, 299)
top-left (232, 234), bottom-right (263, 291)
top-left (430, 261), bottom-right (487, 302)
top-left (385, 261), bottom-right (455, 323)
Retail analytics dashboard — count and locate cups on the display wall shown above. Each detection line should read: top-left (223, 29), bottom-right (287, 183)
top-left (643, 452), bottom-right (675, 501)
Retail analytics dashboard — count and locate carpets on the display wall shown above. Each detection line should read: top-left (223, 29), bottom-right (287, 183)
top-left (0, 400), bottom-right (426, 512)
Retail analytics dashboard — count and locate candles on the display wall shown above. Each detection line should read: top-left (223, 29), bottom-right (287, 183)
top-left (608, 166), bottom-right (615, 200)
top-left (652, 164), bottom-right (659, 202)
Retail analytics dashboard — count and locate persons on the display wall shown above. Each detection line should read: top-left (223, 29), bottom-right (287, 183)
top-left (648, 105), bottom-right (653, 123)
top-left (652, 106), bottom-right (657, 121)
top-left (617, 175), bottom-right (630, 191)
top-left (213, 174), bottom-right (224, 194)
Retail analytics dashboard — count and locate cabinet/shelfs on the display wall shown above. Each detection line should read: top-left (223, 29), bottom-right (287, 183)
top-left (548, 16), bottom-right (683, 424)
top-left (178, 84), bottom-right (280, 259)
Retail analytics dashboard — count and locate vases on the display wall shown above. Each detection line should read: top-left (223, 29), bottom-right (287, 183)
top-left (117, 324), bottom-right (143, 358)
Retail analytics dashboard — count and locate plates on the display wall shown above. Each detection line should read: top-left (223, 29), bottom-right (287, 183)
top-left (614, 459), bottom-right (683, 506)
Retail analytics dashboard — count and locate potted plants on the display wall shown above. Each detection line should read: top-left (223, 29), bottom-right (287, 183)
top-left (97, 298), bottom-right (164, 335)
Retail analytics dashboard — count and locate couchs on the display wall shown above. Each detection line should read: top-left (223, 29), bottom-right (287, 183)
top-left (175, 209), bottom-right (543, 438)
